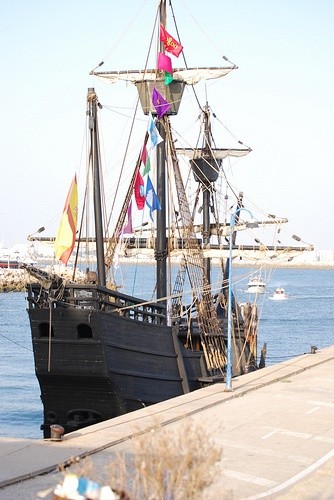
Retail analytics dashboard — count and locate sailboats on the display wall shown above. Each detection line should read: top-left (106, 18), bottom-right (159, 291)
top-left (18, 0), bottom-right (314, 440)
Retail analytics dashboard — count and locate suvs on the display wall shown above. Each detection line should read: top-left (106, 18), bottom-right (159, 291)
top-left (0, 257), bottom-right (37, 269)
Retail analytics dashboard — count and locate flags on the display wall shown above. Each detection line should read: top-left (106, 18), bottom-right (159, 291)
top-left (159, 24), bottom-right (183, 58)
top-left (146, 177), bottom-right (162, 223)
top-left (54, 175), bottom-right (78, 267)
top-left (152, 87), bottom-right (171, 119)
top-left (122, 204), bottom-right (133, 234)
top-left (158, 53), bottom-right (173, 85)
top-left (140, 145), bottom-right (151, 177)
top-left (133, 170), bottom-right (146, 210)
top-left (146, 110), bottom-right (164, 150)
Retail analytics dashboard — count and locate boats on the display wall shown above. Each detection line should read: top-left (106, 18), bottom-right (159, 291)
top-left (247, 277), bottom-right (267, 294)
top-left (273, 288), bottom-right (287, 300)
